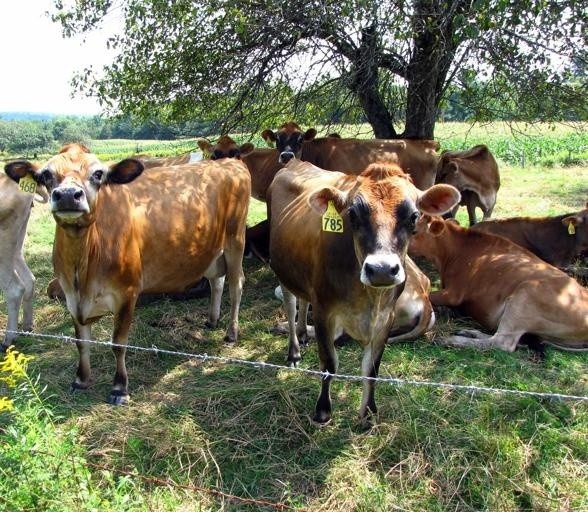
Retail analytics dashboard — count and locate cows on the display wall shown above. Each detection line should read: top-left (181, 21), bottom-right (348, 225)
top-left (472, 212), bottom-right (586, 285)
top-left (5, 143), bottom-right (252, 404)
top-left (1, 171), bottom-right (50, 350)
top-left (130, 148), bottom-right (202, 168)
top-left (197, 133), bottom-right (363, 258)
top-left (410, 212), bottom-right (587, 354)
top-left (275, 252), bottom-right (436, 347)
top-left (268, 157), bottom-right (461, 425)
top-left (436, 144), bottom-right (499, 225)
top-left (260, 123), bottom-right (439, 191)
top-left (47, 223), bottom-right (255, 303)
